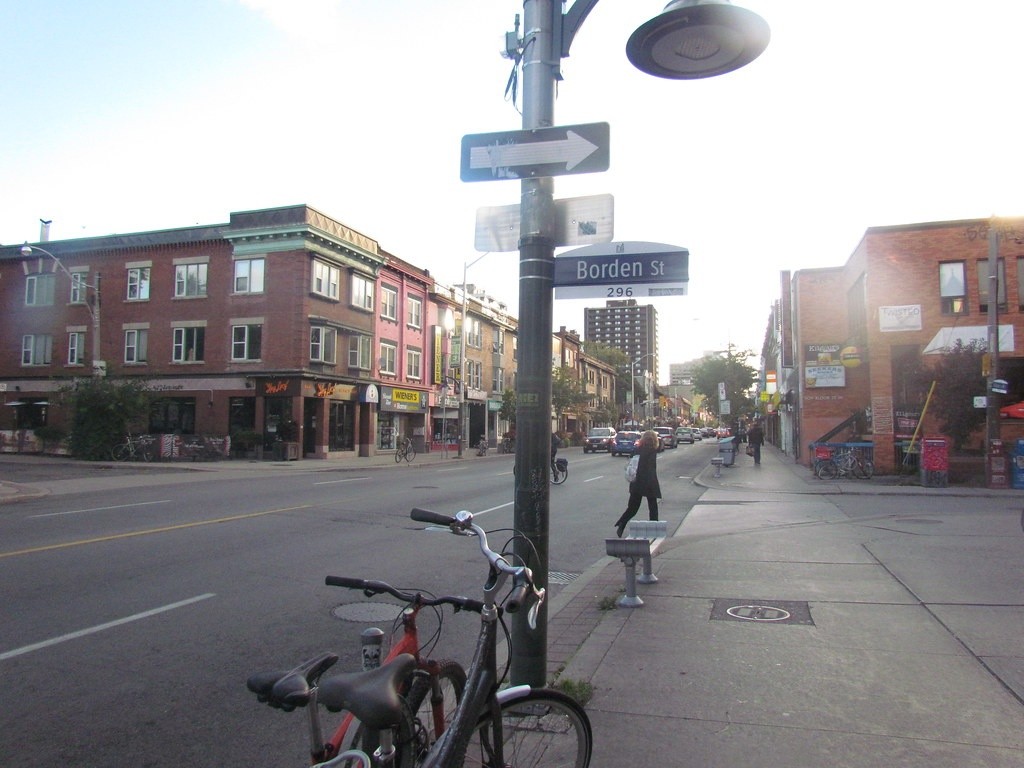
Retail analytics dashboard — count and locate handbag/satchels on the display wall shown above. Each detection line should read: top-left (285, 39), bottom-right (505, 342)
top-left (624, 447), bottom-right (640, 482)
top-left (746, 441), bottom-right (755, 457)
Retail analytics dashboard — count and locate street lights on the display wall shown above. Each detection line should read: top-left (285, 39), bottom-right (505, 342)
top-left (631, 353), bottom-right (658, 430)
top-left (675, 383), bottom-right (690, 426)
top-left (511, 0), bottom-right (769, 712)
top-left (20, 242), bottom-right (100, 375)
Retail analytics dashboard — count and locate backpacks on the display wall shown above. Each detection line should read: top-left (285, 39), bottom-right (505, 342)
top-left (557, 458), bottom-right (568, 472)
top-left (552, 433), bottom-right (561, 448)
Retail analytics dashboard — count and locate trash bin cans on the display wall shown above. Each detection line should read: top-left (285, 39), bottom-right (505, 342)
top-left (718, 436), bottom-right (735, 465)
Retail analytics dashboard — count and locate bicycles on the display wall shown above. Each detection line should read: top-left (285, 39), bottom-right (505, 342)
top-left (247, 574), bottom-right (503, 767)
top-left (111, 436), bottom-right (153, 462)
top-left (814, 446), bottom-right (874, 479)
top-left (395, 437), bottom-right (416, 463)
top-left (513, 457), bottom-right (568, 485)
top-left (315, 508), bottom-right (594, 768)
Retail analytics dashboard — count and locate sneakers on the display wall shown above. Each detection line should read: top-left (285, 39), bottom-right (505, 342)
top-left (555, 471), bottom-right (559, 482)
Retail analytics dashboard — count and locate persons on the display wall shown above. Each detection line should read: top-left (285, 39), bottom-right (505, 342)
top-left (551, 432), bottom-right (560, 482)
top-left (615, 431), bottom-right (662, 538)
top-left (747, 423), bottom-right (764, 465)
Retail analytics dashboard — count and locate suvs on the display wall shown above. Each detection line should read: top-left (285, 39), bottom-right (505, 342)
top-left (583, 426), bottom-right (618, 454)
top-left (675, 427), bottom-right (694, 444)
top-left (652, 427), bottom-right (679, 449)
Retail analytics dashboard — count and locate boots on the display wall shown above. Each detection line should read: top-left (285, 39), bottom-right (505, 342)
top-left (615, 508), bottom-right (636, 537)
top-left (649, 512), bottom-right (658, 521)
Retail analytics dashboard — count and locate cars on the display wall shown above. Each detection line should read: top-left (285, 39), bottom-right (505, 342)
top-left (706, 428), bottom-right (731, 439)
top-left (641, 432), bottom-right (665, 453)
top-left (611, 430), bottom-right (641, 456)
top-left (691, 428), bottom-right (703, 440)
top-left (700, 429), bottom-right (709, 438)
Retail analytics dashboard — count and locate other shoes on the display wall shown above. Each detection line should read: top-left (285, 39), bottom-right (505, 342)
top-left (755, 462), bottom-right (760, 465)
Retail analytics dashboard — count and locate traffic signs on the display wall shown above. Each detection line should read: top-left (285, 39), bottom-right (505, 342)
top-left (552, 241), bottom-right (689, 300)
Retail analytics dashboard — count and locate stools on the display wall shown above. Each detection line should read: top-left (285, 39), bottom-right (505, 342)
top-left (710, 457), bottom-right (723, 477)
top-left (605, 520), bottom-right (668, 606)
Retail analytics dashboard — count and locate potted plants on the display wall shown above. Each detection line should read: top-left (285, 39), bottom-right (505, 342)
top-left (276, 420), bottom-right (298, 461)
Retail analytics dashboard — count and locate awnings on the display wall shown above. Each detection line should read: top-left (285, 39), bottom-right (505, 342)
top-left (921, 325), bottom-right (1014, 355)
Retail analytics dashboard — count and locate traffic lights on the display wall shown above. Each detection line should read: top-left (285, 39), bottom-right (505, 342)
top-left (663, 402), bottom-right (666, 409)
